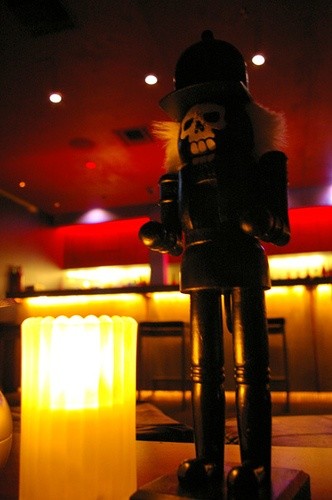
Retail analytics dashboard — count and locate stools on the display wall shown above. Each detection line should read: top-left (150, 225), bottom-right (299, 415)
top-left (262, 318), bottom-right (290, 411)
top-left (136, 319), bottom-right (189, 411)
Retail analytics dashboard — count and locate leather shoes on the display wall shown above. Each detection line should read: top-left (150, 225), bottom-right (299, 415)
top-left (226, 458), bottom-right (272, 499)
top-left (178, 457), bottom-right (225, 491)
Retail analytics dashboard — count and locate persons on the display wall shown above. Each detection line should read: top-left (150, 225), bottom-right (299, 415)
top-left (140, 96), bottom-right (290, 493)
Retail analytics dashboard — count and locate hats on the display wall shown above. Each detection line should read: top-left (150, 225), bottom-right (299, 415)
top-left (159, 29), bottom-right (253, 123)
